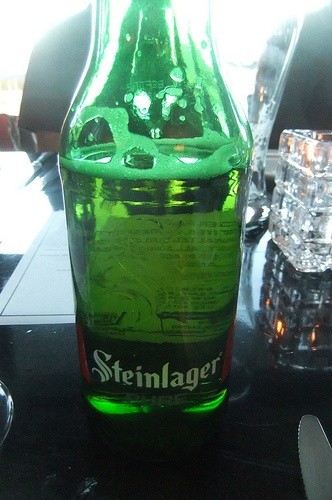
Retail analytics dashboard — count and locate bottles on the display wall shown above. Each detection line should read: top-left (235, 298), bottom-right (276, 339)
top-left (57, 0), bottom-right (254, 415)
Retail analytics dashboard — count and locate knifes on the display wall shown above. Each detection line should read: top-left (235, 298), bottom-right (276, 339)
top-left (297, 414), bottom-right (332, 500)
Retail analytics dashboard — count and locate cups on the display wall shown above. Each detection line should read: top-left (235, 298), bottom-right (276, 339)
top-left (241, 131), bottom-right (271, 245)
top-left (259, 129), bottom-right (332, 370)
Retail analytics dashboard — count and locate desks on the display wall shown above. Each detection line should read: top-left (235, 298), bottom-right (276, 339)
top-left (1, 204), bottom-right (332, 500)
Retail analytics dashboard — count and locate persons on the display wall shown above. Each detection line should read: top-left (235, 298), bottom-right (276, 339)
top-left (18, 0), bottom-right (203, 154)
